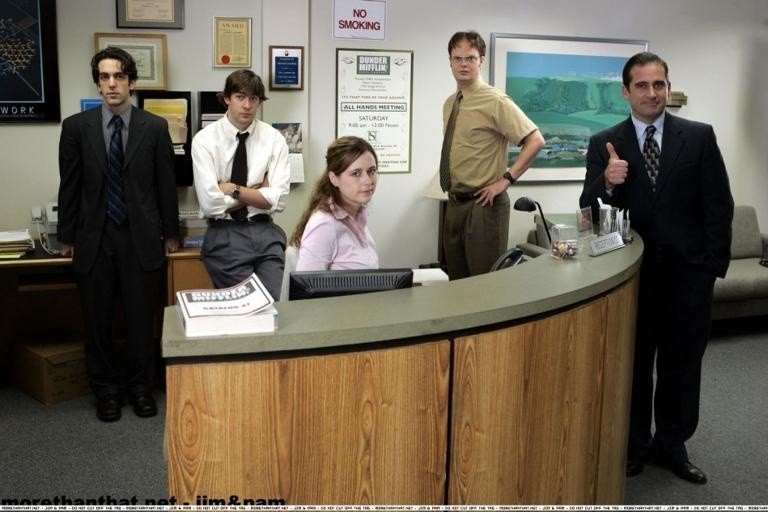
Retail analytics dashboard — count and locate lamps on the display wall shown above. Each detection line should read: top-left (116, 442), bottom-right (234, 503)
top-left (514, 197), bottom-right (551, 245)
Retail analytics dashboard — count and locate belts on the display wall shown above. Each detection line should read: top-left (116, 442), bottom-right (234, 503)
top-left (208, 214), bottom-right (265, 224)
top-left (448, 192), bottom-right (481, 201)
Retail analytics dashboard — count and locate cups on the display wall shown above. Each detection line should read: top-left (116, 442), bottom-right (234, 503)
top-left (599, 206), bottom-right (619, 236)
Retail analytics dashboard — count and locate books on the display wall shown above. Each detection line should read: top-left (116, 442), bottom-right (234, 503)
top-left (177, 206), bottom-right (210, 247)
top-left (176, 273), bottom-right (278, 337)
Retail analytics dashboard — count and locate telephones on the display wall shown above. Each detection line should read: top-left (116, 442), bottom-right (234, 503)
top-left (31, 202), bottom-right (58, 226)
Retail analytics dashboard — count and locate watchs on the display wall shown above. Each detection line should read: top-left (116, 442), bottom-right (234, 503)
top-left (231, 183), bottom-right (240, 199)
top-left (502, 172), bottom-right (515, 185)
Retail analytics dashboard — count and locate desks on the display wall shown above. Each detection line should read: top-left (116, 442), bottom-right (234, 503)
top-left (0, 238), bottom-right (217, 305)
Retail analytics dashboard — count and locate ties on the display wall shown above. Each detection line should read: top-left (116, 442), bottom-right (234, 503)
top-left (230, 132), bottom-right (248, 221)
top-left (106, 115), bottom-right (127, 224)
top-left (439, 91), bottom-right (462, 192)
top-left (640, 125), bottom-right (660, 192)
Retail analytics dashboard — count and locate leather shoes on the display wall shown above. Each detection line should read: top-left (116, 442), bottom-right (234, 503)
top-left (667, 461), bottom-right (706, 484)
top-left (132, 392), bottom-right (157, 417)
top-left (96, 394), bottom-right (120, 422)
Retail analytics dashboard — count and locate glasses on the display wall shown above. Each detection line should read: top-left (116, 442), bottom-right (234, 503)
top-left (453, 56), bottom-right (480, 64)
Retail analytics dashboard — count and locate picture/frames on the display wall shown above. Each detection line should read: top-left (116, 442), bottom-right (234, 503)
top-left (335, 48), bottom-right (413, 174)
top-left (94, 32), bottom-right (168, 90)
top-left (1, 1), bottom-right (61, 123)
top-left (115, 0), bottom-right (186, 30)
top-left (213, 16), bottom-right (253, 69)
top-left (268, 45), bottom-right (304, 90)
top-left (488, 32), bottom-right (649, 186)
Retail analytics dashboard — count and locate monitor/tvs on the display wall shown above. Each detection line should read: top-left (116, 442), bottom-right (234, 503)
top-left (289, 269), bottom-right (412, 302)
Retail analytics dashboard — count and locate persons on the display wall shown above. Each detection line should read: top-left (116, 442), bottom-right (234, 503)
top-left (439, 32), bottom-right (545, 280)
top-left (581, 53), bottom-right (735, 484)
top-left (291, 137), bottom-right (379, 270)
top-left (57, 48), bottom-right (180, 422)
top-left (190, 69), bottom-right (291, 302)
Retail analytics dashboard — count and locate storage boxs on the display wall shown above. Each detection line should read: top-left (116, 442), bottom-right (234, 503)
top-left (9, 336), bottom-right (96, 406)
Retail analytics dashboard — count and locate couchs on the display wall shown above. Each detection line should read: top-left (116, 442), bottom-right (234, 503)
top-left (709, 205), bottom-right (768, 322)
